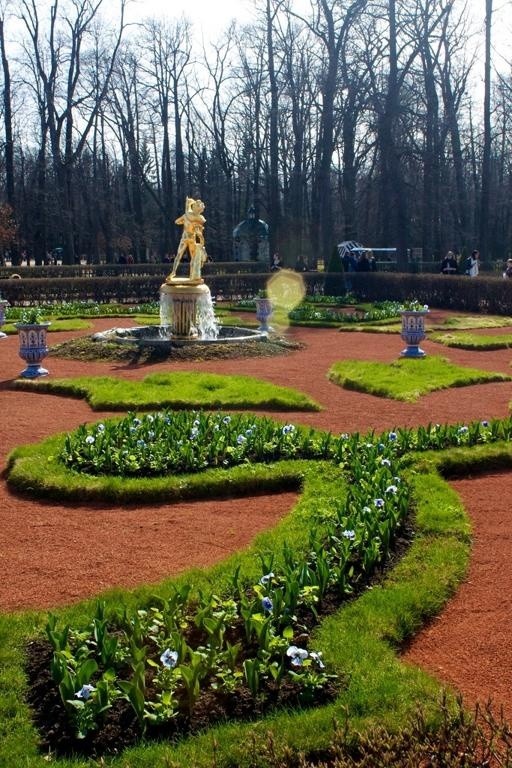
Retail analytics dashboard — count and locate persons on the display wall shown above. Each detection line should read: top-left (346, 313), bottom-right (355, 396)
top-left (118, 252), bottom-right (134, 264)
top-left (295, 251), bottom-right (377, 296)
top-left (270, 253), bottom-right (283, 272)
top-left (165, 199), bottom-right (206, 280)
top-left (163, 253), bottom-right (213, 263)
top-left (45, 250), bottom-right (58, 265)
top-left (465, 249), bottom-right (480, 276)
top-left (439, 251), bottom-right (458, 275)
top-left (502, 258), bottom-right (512, 280)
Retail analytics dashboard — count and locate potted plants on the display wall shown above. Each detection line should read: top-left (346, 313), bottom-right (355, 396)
top-left (0, 298), bottom-right (11, 338)
top-left (13, 306), bottom-right (51, 378)
top-left (397, 300), bottom-right (430, 357)
top-left (253, 290), bottom-right (274, 332)
